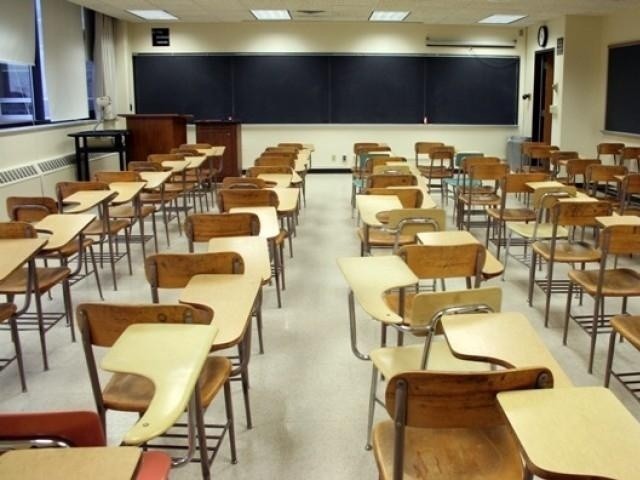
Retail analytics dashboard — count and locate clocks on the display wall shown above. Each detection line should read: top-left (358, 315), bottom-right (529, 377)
top-left (535, 22), bottom-right (550, 50)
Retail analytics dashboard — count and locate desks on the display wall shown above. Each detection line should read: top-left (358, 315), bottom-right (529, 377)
top-left (0, 237), bottom-right (47, 318)
top-left (292, 143), bottom-right (314, 172)
top-left (176, 272), bottom-right (265, 377)
top-left (557, 192), bottom-right (600, 202)
top-left (592, 212), bottom-right (639, 228)
top-left (439, 310), bottom-right (574, 389)
top-left (107, 179), bottom-right (147, 202)
top-left (56, 189), bottom-right (120, 244)
top-left (494, 384), bottom-right (639, 479)
top-left (290, 167), bottom-right (302, 184)
top-left (100, 323), bottom-right (220, 470)
top-left (183, 155), bottom-right (207, 169)
top-left (207, 235), bottom-right (273, 287)
top-left (228, 206), bottom-right (280, 241)
top-left (355, 194), bottom-right (404, 229)
top-left (261, 188), bottom-right (300, 213)
top-left (384, 185), bottom-right (438, 209)
top-left (385, 162), bottom-right (430, 193)
top-left (29, 212), bottom-right (98, 279)
top-left (524, 180), bottom-right (580, 193)
top-left (2, 446), bottom-right (172, 480)
top-left (66, 129), bottom-right (133, 180)
top-left (136, 170), bottom-right (177, 190)
top-left (194, 145), bottom-right (226, 173)
top-left (333, 253), bottom-right (420, 362)
top-left (256, 173), bottom-right (293, 188)
top-left (414, 229), bottom-right (505, 282)
top-left (161, 160), bottom-right (191, 174)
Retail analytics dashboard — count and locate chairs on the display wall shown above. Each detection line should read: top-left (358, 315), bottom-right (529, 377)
top-left (223, 176), bottom-right (266, 189)
top-left (178, 143), bottom-right (219, 208)
top-left (76, 301), bottom-right (238, 479)
top-left (183, 211), bottom-right (265, 355)
top-left (373, 165), bottom-right (411, 173)
top-left (604, 314), bottom-right (639, 403)
top-left (143, 250), bottom-right (254, 430)
top-left (484, 171), bottom-right (553, 260)
top-left (148, 153), bottom-right (197, 217)
top-left (356, 187), bottom-right (424, 257)
top-left (277, 142), bottom-right (304, 151)
top-left (1, 408), bottom-right (109, 452)
top-left (414, 141), bottom-right (445, 171)
top-left (460, 163), bottom-right (511, 235)
top-left (253, 156), bottom-right (295, 169)
top-left (0, 220), bottom-right (77, 371)
top-left (603, 313), bottom-right (640, 404)
top-left (168, 147), bottom-right (211, 213)
top-left (93, 170), bottom-right (158, 265)
top-left (380, 243), bottom-right (488, 382)
top-left (6, 195), bottom-right (105, 328)
top-left (54, 181), bottom-right (133, 292)
top-left (597, 141), bottom-right (627, 165)
top-left (389, 208), bottom-right (446, 294)
top-left (127, 160), bottom-right (182, 248)
top-left (565, 158), bottom-right (602, 196)
top-left (500, 186), bottom-right (577, 282)
top-left (618, 146), bottom-right (639, 172)
top-left (261, 152), bottom-right (298, 159)
top-left (527, 200), bottom-right (613, 329)
top-left (264, 146), bottom-right (301, 155)
top-left (365, 180), bottom-right (418, 188)
top-left (365, 285), bottom-right (503, 452)
top-left (365, 174), bottom-right (419, 189)
top-left (245, 165), bottom-right (294, 177)
top-left (371, 366), bottom-right (555, 479)
top-left (561, 224), bottom-right (640, 374)
top-left (422, 145), bottom-right (455, 191)
top-left (0, 302), bottom-right (26, 393)
top-left (215, 188), bottom-right (286, 309)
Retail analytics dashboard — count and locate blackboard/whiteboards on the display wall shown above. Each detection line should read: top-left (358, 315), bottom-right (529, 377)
top-left (132, 52), bottom-right (522, 128)
top-left (601, 39), bottom-right (639, 139)
